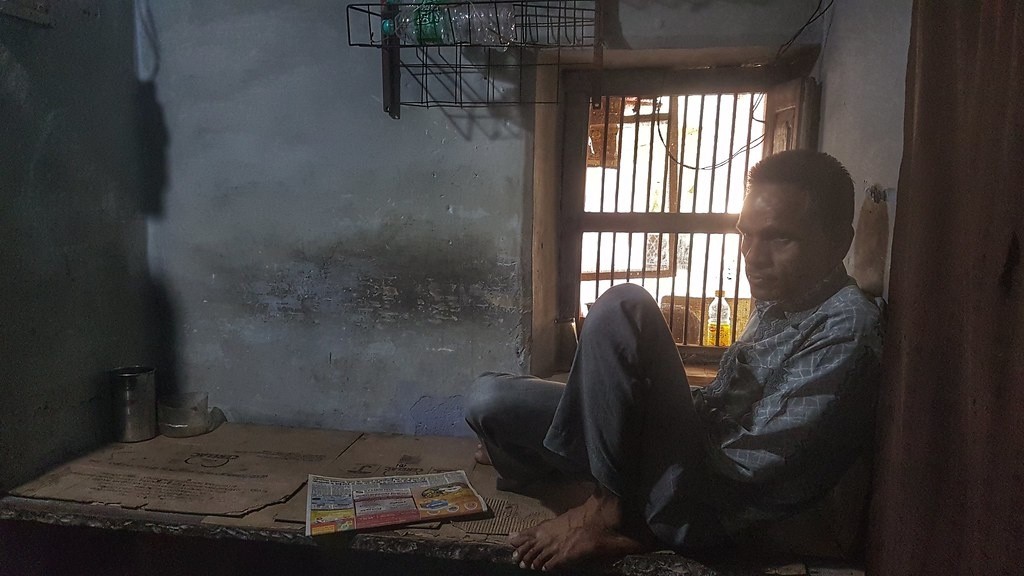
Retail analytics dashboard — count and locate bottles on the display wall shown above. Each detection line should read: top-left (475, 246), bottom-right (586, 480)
top-left (383, 0), bottom-right (515, 46)
top-left (708, 290), bottom-right (730, 346)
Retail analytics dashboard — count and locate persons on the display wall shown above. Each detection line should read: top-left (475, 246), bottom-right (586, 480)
top-left (464, 149), bottom-right (886, 570)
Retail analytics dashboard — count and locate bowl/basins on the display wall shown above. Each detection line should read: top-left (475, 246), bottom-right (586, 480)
top-left (157, 391), bottom-right (208, 437)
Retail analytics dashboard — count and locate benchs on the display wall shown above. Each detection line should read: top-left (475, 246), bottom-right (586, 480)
top-left (0, 423), bottom-right (639, 576)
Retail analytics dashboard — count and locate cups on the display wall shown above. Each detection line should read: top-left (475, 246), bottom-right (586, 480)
top-left (110, 367), bottom-right (156, 443)
